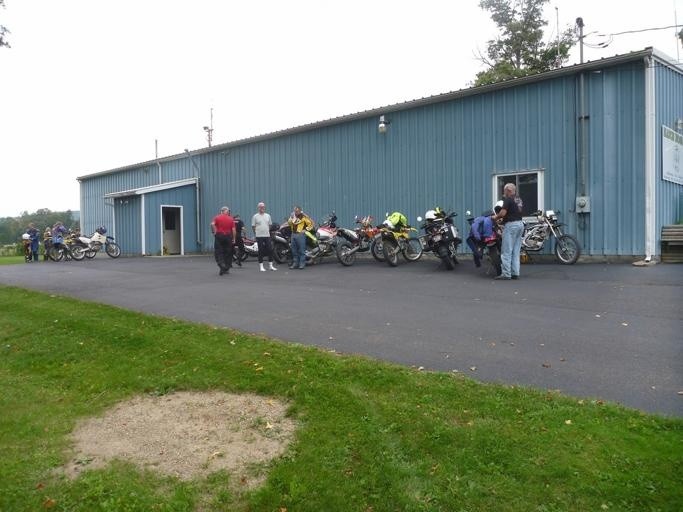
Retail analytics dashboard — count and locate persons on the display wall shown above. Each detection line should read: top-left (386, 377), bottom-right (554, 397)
top-left (25, 222), bottom-right (41, 262)
top-left (209, 205), bottom-right (236, 276)
top-left (51, 223), bottom-right (55, 246)
top-left (285, 206), bottom-right (314, 269)
top-left (487, 182), bottom-right (524, 280)
top-left (43, 226), bottom-right (51, 261)
top-left (232, 212), bottom-right (245, 267)
top-left (250, 200), bottom-right (278, 272)
top-left (55, 220), bottom-right (69, 261)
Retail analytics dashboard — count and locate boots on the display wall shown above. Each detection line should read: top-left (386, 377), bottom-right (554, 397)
top-left (259, 263), bottom-right (267, 272)
top-left (268, 261), bottom-right (277, 271)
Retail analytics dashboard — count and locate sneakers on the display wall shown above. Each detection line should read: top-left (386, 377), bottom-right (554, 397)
top-left (494, 273), bottom-right (518, 281)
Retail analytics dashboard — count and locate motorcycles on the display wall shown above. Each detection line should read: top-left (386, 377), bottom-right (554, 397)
top-left (521, 208), bottom-right (580, 263)
top-left (232, 210), bottom-right (423, 266)
top-left (47, 225), bottom-right (122, 261)
top-left (463, 205), bottom-right (503, 277)
top-left (416, 207), bottom-right (462, 271)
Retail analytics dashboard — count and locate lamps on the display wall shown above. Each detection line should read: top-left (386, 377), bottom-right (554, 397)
top-left (378, 116), bottom-right (390, 131)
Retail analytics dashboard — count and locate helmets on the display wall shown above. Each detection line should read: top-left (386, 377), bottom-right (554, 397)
top-left (494, 200), bottom-right (504, 214)
top-left (424, 210), bottom-right (437, 220)
